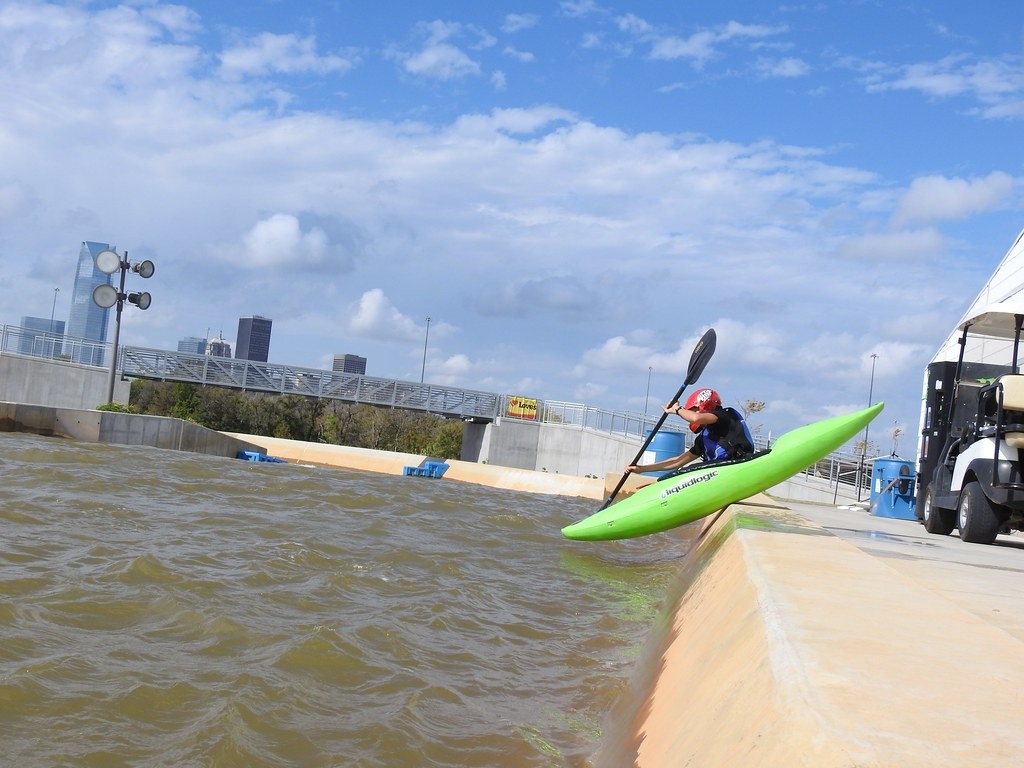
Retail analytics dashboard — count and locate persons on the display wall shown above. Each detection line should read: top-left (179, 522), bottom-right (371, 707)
top-left (624, 388), bottom-right (755, 490)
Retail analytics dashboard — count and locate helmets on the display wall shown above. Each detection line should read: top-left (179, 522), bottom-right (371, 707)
top-left (685, 389), bottom-right (722, 433)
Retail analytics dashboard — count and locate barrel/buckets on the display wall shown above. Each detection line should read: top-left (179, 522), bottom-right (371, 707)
top-left (640, 430), bottom-right (686, 476)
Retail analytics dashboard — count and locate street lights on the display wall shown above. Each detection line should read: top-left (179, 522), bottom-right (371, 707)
top-left (46, 287), bottom-right (61, 357)
top-left (643, 366), bottom-right (653, 435)
top-left (421, 317), bottom-right (432, 383)
top-left (862, 354), bottom-right (880, 455)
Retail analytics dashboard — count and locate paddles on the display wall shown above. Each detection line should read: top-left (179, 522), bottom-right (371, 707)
top-left (596, 328), bottom-right (717, 511)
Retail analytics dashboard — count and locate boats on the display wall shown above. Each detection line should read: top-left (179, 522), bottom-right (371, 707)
top-left (561, 402), bottom-right (886, 543)
